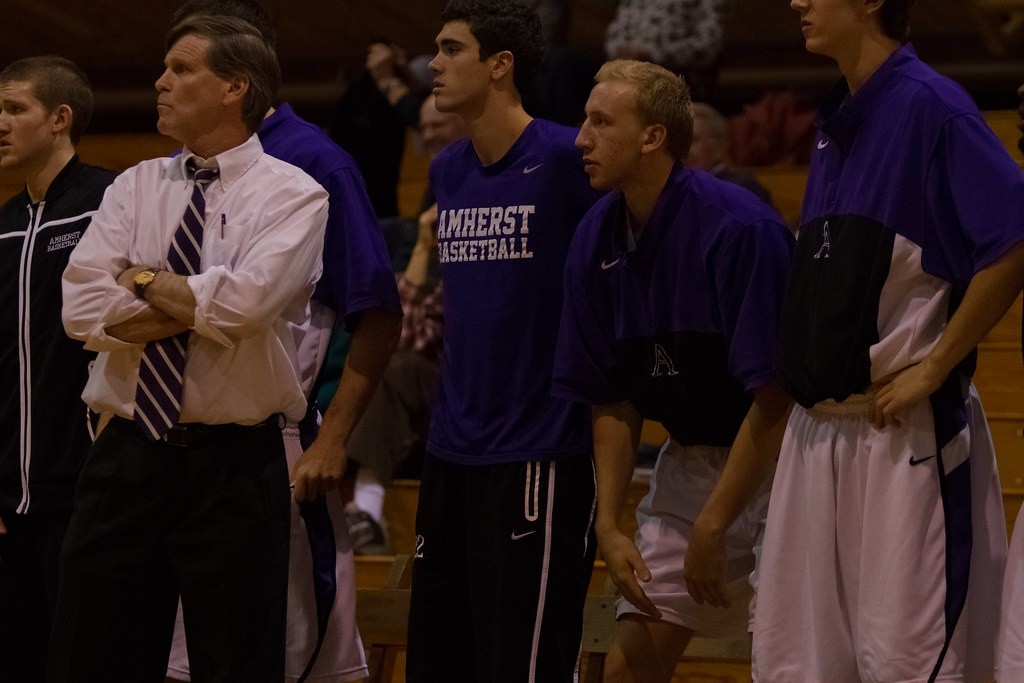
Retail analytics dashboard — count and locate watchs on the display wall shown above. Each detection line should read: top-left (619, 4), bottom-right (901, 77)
top-left (134, 267), bottom-right (161, 299)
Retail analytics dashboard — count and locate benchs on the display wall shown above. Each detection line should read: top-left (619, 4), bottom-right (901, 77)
top-left (0, 104), bottom-right (1024, 683)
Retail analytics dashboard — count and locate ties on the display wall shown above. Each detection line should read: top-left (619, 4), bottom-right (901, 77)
top-left (135, 156), bottom-right (220, 441)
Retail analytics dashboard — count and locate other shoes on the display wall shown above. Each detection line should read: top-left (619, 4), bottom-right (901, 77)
top-left (342, 502), bottom-right (392, 557)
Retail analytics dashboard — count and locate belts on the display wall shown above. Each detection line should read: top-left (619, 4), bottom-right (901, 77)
top-left (109, 412), bottom-right (280, 443)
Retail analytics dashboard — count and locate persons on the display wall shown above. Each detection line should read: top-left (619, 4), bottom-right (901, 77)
top-left (166, 0), bottom-right (405, 683)
top-left (406, 0), bottom-right (603, 683)
top-left (45, 15), bottom-right (330, 683)
top-left (552, 60), bottom-right (794, 683)
top-left (750, 0), bottom-right (1024, 683)
top-left (334, 40), bottom-right (430, 216)
top-left (0, 56), bottom-right (116, 683)
top-left (689, 103), bottom-right (774, 206)
top-left (345, 95), bottom-right (467, 556)
top-left (552, 0), bottom-right (734, 128)
top-left (995, 84), bottom-right (1024, 683)
